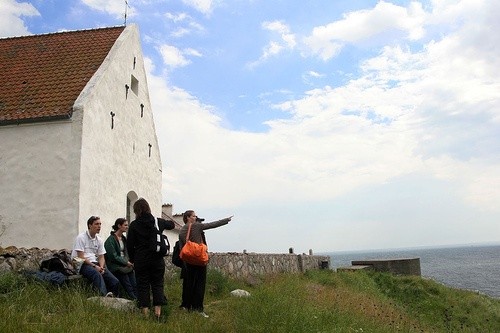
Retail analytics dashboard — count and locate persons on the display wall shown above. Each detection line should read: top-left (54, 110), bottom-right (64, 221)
top-left (126, 198), bottom-right (175, 322)
top-left (179, 210), bottom-right (234, 317)
top-left (103, 218), bottom-right (137, 299)
top-left (71, 216), bottom-right (120, 298)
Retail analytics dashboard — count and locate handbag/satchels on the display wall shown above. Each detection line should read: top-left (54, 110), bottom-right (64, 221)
top-left (179, 223), bottom-right (208, 266)
top-left (40, 258), bottom-right (76, 275)
top-left (155, 216), bottom-right (170, 256)
top-left (172, 240), bottom-right (182, 269)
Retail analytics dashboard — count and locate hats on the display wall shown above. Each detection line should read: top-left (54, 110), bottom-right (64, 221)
top-left (196, 216), bottom-right (204, 221)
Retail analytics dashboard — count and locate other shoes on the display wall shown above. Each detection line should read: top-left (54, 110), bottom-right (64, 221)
top-left (199, 311), bottom-right (209, 318)
top-left (107, 292), bottom-right (113, 297)
